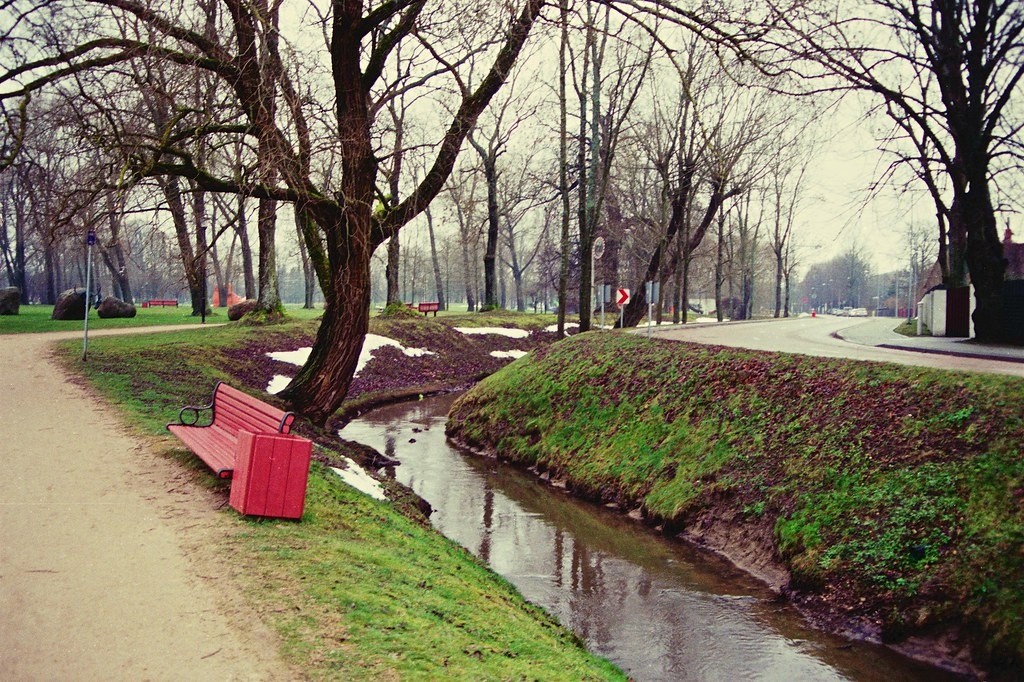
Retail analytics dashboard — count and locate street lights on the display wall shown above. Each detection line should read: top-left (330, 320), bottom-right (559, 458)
top-left (790, 243), bottom-right (823, 314)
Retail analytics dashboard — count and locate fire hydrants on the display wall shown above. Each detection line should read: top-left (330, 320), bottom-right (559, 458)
top-left (812, 308), bottom-right (817, 317)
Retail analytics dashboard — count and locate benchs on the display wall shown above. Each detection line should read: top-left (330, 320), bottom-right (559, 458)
top-left (419, 302), bottom-right (439, 317)
top-left (149, 300), bottom-right (177, 309)
top-left (167, 380), bottom-right (294, 478)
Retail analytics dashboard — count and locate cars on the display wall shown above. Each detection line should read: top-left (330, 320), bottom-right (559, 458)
top-left (827, 306), bottom-right (869, 317)
top-left (875, 306), bottom-right (892, 316)
